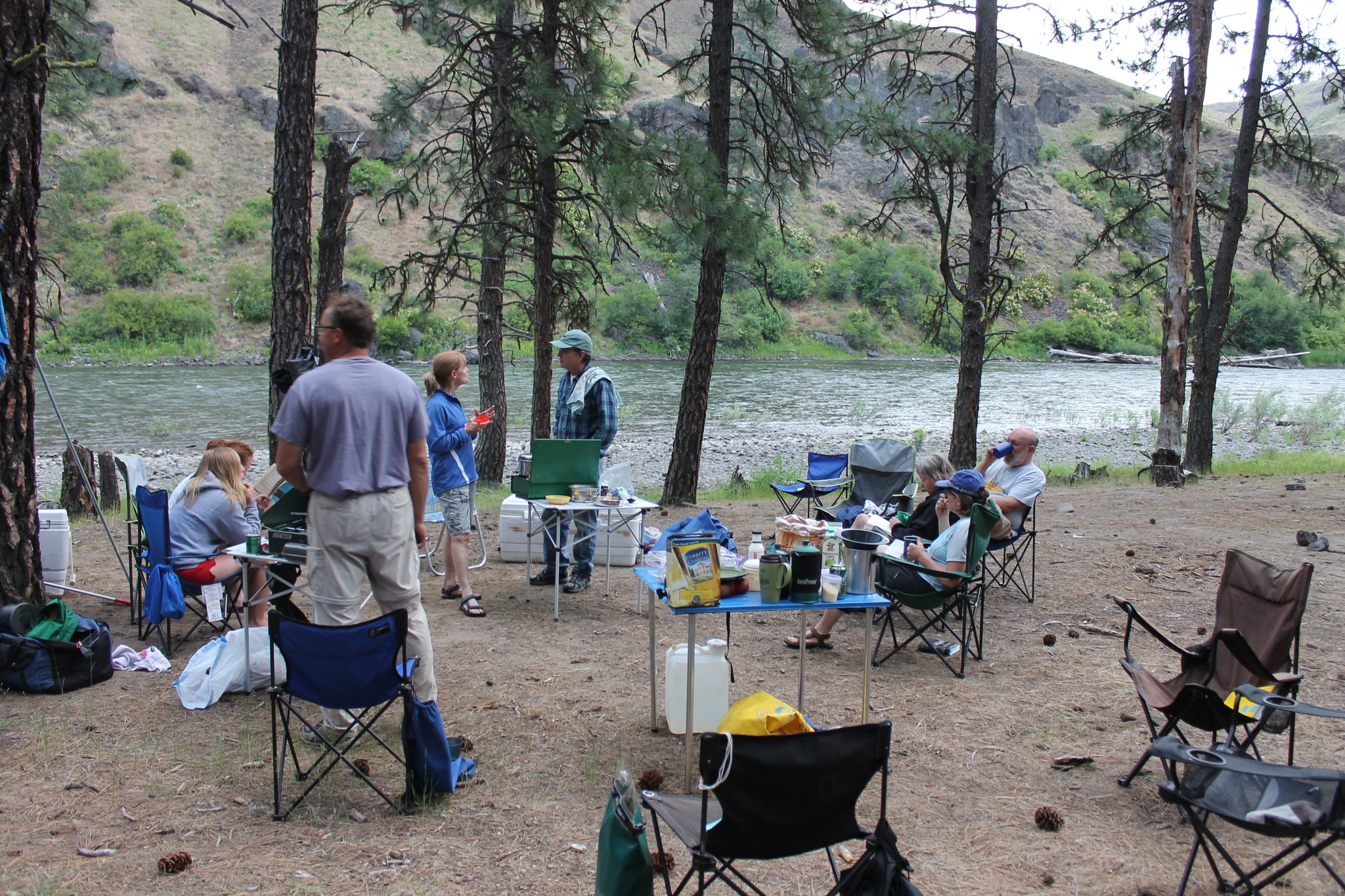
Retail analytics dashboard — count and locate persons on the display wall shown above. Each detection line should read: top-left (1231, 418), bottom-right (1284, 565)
top-left (973, 427), bottom-right (1046, 540)
top-left (784, 468), bottom-right (989, 649)
top-left (422, 350), bottom-right (496, 617)
top-left (169, 439), bottom-right (253, 511)
top-left (169, 446), bottom-right (269, 627)
top-left (529, 329), bottom-right (619, 593)
top-left (270, 292), bottom-right (441, 744)
top-left (842, 453), bottom-right (957, 568)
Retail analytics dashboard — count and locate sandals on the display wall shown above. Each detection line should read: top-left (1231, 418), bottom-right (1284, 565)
top-left (784, 627), bottom-right (834, 650)
top-left (459, 594), bottom-right (486, 618)
top-left (440, 583), bottom-right (482, 600)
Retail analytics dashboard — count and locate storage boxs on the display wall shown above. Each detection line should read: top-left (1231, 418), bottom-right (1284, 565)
top-left (37, 510), bottom-right (77, 599)
top-left (499, 494), bottom-right (642, 567)
top-left (259, 484), bottom-right (309, 554)
top-left (511, 438), bottom-right (601, 500)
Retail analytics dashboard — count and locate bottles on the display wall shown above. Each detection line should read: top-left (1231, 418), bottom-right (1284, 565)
top-left (748, 530), bottom-right (765, 559)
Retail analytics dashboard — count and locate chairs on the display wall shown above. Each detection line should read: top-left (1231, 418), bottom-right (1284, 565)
top-left (796, 437), bottom-right (918, 528)
top-left (770, 450), bottom-right (848, 520)
top-left (640, 719), bottom-right (896, 896)
top-left (979, 484), bottom-right (1045, 604)
top-left (1144, 684), bottom-right (1345, 896)
top-left (114, 451), bottom-right (256, 660)
top-left (1106, 547), bottom-right (1314, 820)
top-left (872, 500), bottom-right (1003, 679)
top-left (264, 609), bottom-right (419, 821)
top-left (407, 449), bottom-right (487, 576)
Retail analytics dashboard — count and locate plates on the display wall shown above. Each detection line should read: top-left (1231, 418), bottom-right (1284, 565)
top-left (721, 566), bottom-right (748, 582)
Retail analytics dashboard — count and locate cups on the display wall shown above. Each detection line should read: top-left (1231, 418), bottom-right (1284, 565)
top-left (903, 536), bottom-right (918, 555)
top-left (475, 415), bottom-right (489, 427)
top-left (897, 511), bottom-right (911, 524)
top-left (821, 573), bottom-right (842, 603)
top-left (759, 554), bottom-right (792, 604)
top-left (993, 442), bottom-right (1012, 459)
top-left (743, 559), bottom-right (760, 591)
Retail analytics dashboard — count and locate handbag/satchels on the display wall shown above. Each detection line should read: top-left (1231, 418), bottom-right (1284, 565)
top-left (0, 613), bottom-right (114, 694)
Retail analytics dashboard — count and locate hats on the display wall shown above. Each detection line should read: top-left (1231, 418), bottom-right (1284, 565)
top-left (934, 469), bottom-right (986, 497)
top-left (548, 329), bottom-right (594, 353)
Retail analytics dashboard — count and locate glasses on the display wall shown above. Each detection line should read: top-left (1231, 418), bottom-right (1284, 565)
top-left (1005, 438), bottom-right (1032, 447)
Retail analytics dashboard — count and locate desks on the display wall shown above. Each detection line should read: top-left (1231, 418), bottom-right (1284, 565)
top-left (630, 567), bottom-right (895, 794)
top-left (514, 495), bottom-right (660, 622)
top-left (222, 527), bottom-right (311, 696)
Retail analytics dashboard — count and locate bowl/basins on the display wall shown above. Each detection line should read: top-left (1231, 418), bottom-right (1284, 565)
top-left (834, 528), bottom-right (890, 551)
top-left (568, 484), bottom-right (599, 503)
top-left (545, 495), bottom-right (571, 505)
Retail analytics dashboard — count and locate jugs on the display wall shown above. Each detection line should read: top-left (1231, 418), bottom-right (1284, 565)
top-left (764, 544), bottom-right (790, 600)
top-left (791, 539), bottom-right (822, 604)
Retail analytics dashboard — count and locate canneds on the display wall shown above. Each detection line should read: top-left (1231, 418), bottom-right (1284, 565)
top-left (246, 534), bottom-right (262, 554)
top-left (829, 564), bottom-right (846, 599)
top-left (601, 485), bottom-right (608, 496)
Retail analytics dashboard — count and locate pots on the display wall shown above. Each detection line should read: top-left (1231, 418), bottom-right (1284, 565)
top-left (518, 454), bottom-right (532, 477)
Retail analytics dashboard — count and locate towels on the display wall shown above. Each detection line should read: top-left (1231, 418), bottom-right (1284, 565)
top-left (566, 366), bottom-right (625, 418)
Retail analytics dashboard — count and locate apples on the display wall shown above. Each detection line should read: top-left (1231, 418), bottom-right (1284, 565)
top-left (720, 578), bottom-right (749, 596)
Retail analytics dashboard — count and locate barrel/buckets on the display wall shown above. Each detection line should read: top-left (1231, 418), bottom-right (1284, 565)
top-left (840, 544), bottom-right (884, 594)
top-left (665, 638), bottom-right (729, 735)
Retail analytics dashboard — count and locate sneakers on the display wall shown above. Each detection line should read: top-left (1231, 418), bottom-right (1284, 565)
top-left (299, 720), bottom-right (361, 749)
top-left (563, 571), bottom-right (591, 593)
top-left (530, 568), bottom-right (568, 586)
